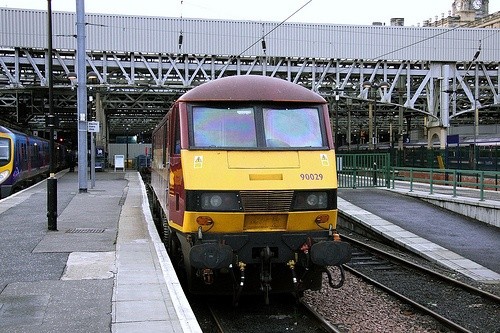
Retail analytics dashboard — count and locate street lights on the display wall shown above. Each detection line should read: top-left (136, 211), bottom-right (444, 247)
top-left (67, 72), bottom-right (98, 178)
top-left (363, 81), bottom-right (391, 185)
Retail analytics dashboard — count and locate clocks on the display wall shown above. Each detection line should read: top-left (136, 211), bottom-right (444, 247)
top-left (454, 2), bottom-right (462, 11)
top-left (473, 0), bottom-right (482, 9)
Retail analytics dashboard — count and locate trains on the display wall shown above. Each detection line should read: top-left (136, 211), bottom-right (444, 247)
top-left (148, 75), bottom-right (353, 308)
top-left (0, 126), bottom-right (70, 198)
